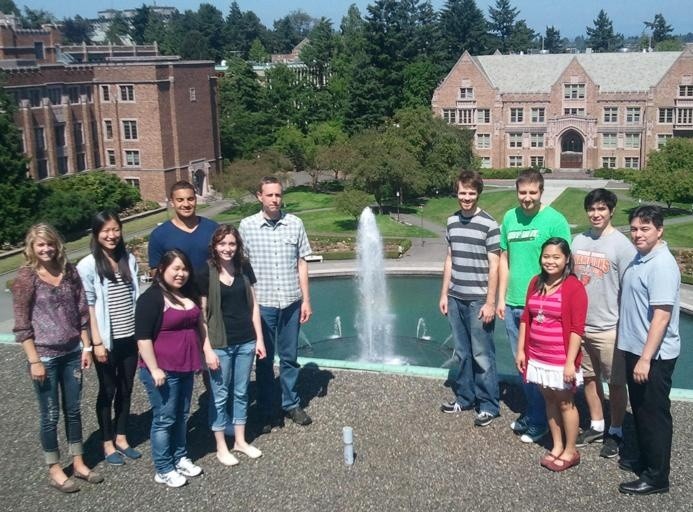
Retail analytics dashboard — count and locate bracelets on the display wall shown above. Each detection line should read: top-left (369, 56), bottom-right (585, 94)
top-left (81, 345), bottom-right (93, 352)
top-left (93, 342), bottom-right (103, 346)
top-left (30, 360), bottom-right (41, 365)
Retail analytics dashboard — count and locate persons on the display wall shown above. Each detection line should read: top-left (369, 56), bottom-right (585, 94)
top-left (146, 179), bottom-right (237, 437)
top-left (566, 187), bottom-right (637, 461)
top-left (435, 169), bottom-right (502, 428)
top-left (235, 174), bottom-right (313, 435)
top-left (614, 202), bottom-right (682, 496)
top-left (133, 249), bottom-right (204, 488)
top-left (511, 236), bottom-right (589, 473)
top-left (494, 167), bottom-right (574, 445)
top-left (191, 224), bottom-right (267, 466)
top-left (13, 223), bottom-right (103, 494)
top-left (398, 244), bottom-right (404, 259)
top-left (74, 210), bottom-right (142, 467)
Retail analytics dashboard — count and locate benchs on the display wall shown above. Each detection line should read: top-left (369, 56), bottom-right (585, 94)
top-left (304, 255), bottom-right (324, 264)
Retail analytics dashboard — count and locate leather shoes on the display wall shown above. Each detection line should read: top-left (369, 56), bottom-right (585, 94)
top-left (620, 458), bottom-right (645, 469)
top-left (619, 478), bottom-right (669, 495)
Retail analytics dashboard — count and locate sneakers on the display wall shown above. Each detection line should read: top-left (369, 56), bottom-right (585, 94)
top-left (520, 425), bottom-right (549, 442)
top-left (103, 451), bottom-right (124, 466)
top-left (154, 468), bottom-right (188, 488)
top-left (510, 415), bottom-right (531, 430)
top-left (49, 475), bottom-right (80, 492)
top-left (116, 444), bottom-right (140, 459)
top-left (176, 457), bottom-right (204, 476)
top-left (441, 399), bottom-right (470, 413)
top-left (255, 412), bottom-right (272, 433)
top-left (546, 451), bottom-right (580, 471)
top-left (600, 432), bottom-right (624, 458)
top-left (74, 469), bottom-right (104, 483)
top-left (216, 451), bottom-right (238, 466)
top-left (284, 408), bottom-right (312, 426)
top-left (234, 443), bottom-right (262, 458)
top-left (475, 412), bottom-right (494, 426)
top-left (576, 427), bottom-right (607, 447)
top-left (540, 446), bottom-right (564, 466)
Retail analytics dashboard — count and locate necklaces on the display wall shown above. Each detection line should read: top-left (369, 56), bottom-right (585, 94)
top-left (536, 295), bottom-right (544, 323)
top-left (222, 272), bottom-right (234, 284)
